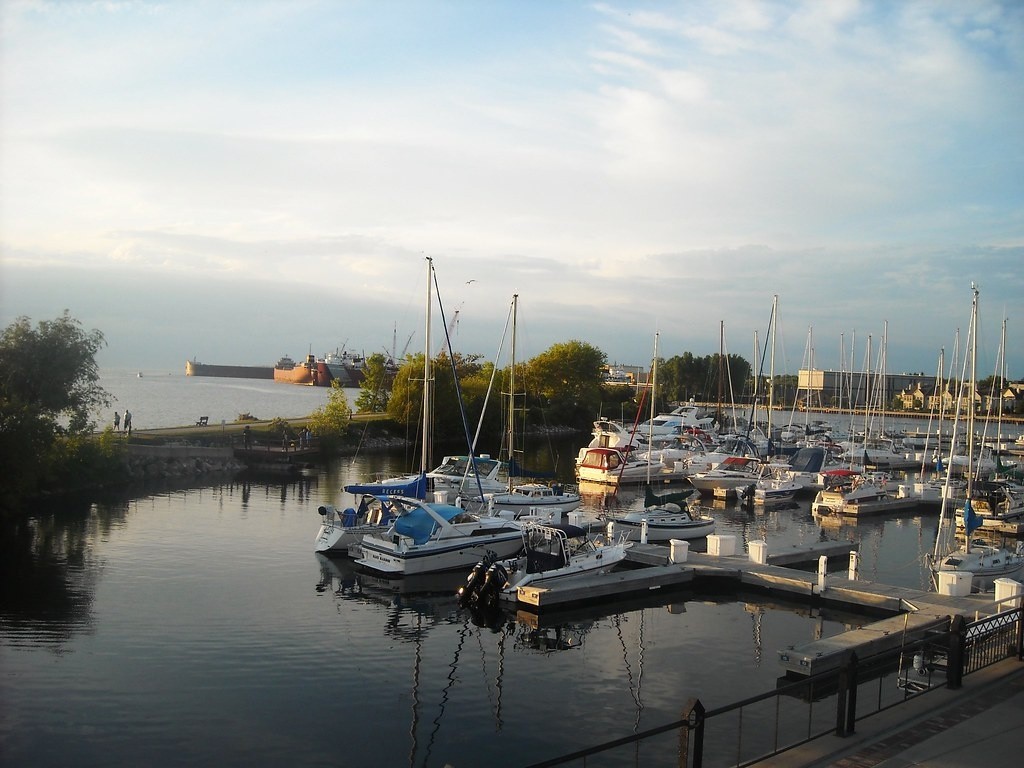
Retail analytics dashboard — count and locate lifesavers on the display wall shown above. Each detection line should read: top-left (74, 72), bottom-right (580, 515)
top-left (548, 480), bottom-right (559, 488)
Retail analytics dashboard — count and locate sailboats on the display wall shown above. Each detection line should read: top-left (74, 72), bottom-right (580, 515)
top-left (212, 198), bottom-right (1024, 606)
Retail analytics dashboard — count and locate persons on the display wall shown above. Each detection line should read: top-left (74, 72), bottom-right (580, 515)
top-left (931, 447), bottom-right (940, 463)
top-left (298, 429), bottom-right (306, 450)
top-left (123, 410), bottom-right (131, 433)
top-left (306, 429), bottom-right (312, 448)
top-left (243, 425), bottom-right (252, 449)
top-left (282, 430), bottom-right (288, 452)
top-left (113, 412), bottom-right (121, 431)
